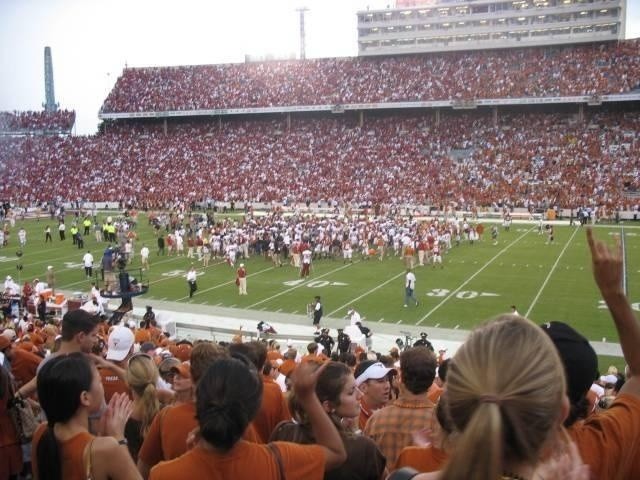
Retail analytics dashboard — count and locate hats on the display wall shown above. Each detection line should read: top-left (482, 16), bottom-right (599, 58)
top-left (389, 347), bottom-right (398, 352)
top-left (540, 321), bottom-right (598, 406)
top-left (355, 362), bottom-right (398, 388)
top-left (270, 359), bottom-right (283, 367)
top-left (600, 375), bottom-right (618, 384)
top-left (140, 343), bottom-right (156, 351)
top-left (337, 329), bottom-right (343, 331)
top-left (0, 328), bottom-right (16, 341)
top-left (106, 327), bottom-right (135, 362)
top-left (437, 346), bottom-right (447, 352)
top-left (170, 361), bottom-right (192, 378)
top-left (0, 335), bottom-right (11, 351)
top-left (321, 329), bottom-right (329, 333)
top-left (159, 358), bottom-right (181, 373)
top-left (421, 332), bottom-right (427, 337)
top-left (347, 309), bottom-right (353, 315)
top-left (80, 300), bottom-right (104, 316)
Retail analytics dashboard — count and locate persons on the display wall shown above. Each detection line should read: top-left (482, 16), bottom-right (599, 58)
top-left (2, 39), bottom-right (639, 479)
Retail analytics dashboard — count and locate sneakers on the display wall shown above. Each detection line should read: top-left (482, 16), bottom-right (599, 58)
top-left (314, 332), bottom-right (320, 334)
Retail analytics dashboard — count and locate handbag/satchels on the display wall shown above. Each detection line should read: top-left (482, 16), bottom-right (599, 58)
top-left (6, 396), bottom-right (46, 444)
